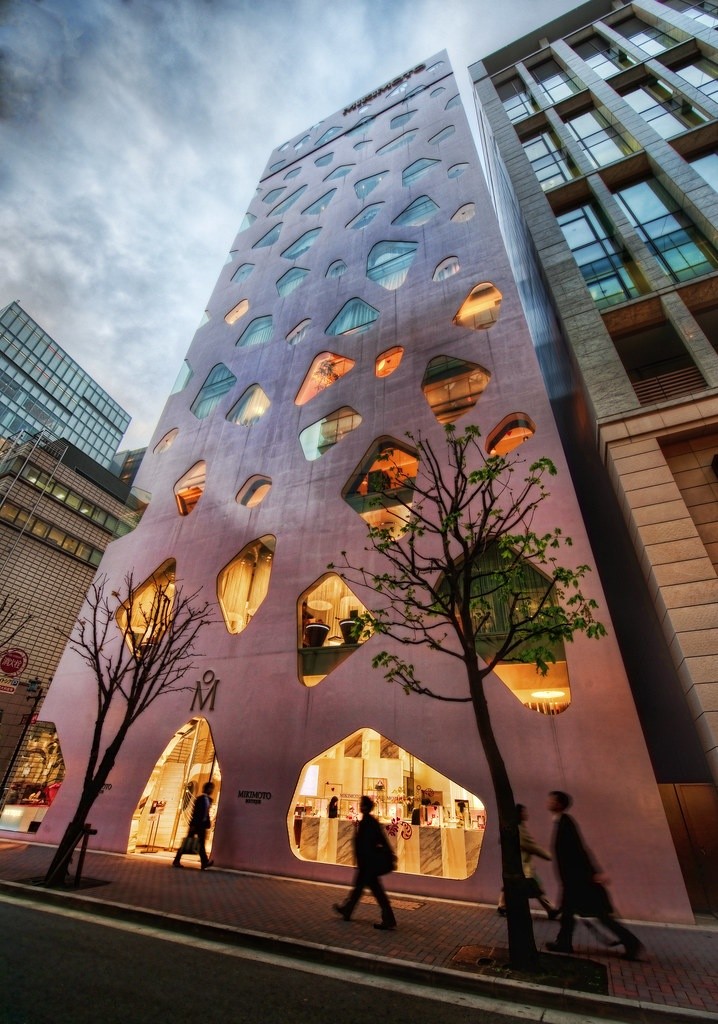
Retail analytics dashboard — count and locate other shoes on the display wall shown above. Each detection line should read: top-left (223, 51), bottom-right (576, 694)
top-left (544, 939), bottom-right (574, 954)
top-left (201, 861), bottom-right (215, 872)
top-left (172, 858), bottom-right (184, 868)
top-left (332, 902), bottom-right (356, 921)
top-left (546, 905), bottom-right (563, 918)
top-left (618, 943), bottom-right (647, 962)
top-left (496, 905), bottom-right (507, 918)
top-left (373, 913), bottom-right (397, 931)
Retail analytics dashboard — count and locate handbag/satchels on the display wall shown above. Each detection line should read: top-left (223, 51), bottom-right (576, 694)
top-left (181, 837), bottom-right (200, 855)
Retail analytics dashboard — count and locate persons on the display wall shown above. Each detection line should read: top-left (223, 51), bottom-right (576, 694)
top-left (172, 781), bottom-right (216, 870)
top-left (409, 798), bottom-right (442, 825)
top-left (330, 792), bottom-right (400, 929)
top-left (326, 796), bottom-right (340, 818)
top-left (454, 800), bottom-right (468, 819)
top-left (496, 802), bottom-right (563, 921)
top-left (544, 789), bottom-right (649, 959)
